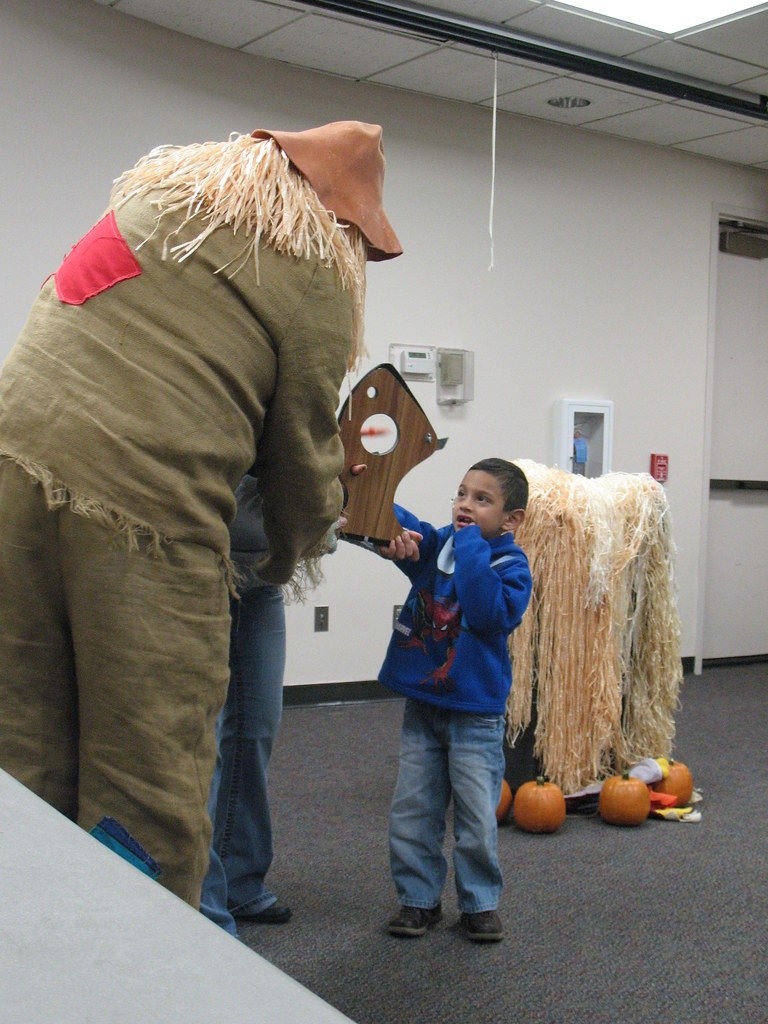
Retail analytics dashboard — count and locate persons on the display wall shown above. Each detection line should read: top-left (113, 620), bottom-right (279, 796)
top-left (385, 457), bottom-right (531, 942)
top-left (0, 120), bottom-right (424, 943)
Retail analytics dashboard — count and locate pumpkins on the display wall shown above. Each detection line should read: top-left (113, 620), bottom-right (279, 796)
top-left (494, 778), bottom-right (512, 823)
top-left (514, 776), bottom-right (566, 834)
top-left (651, 758), bottom-right (693, 808)
top-left (598, 771), bottom-right (651, 826)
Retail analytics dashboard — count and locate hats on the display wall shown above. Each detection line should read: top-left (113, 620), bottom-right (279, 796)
top-left (252, 119), bottom-right (403, 262)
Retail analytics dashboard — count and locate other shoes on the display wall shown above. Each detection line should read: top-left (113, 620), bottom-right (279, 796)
top-left (238, 898), bottom-right (292, 923)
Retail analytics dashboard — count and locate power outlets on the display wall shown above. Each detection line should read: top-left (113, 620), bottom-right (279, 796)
top-left (314, 606), bottom-right (329, 632)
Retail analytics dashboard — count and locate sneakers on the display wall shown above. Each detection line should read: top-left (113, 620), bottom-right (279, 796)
top-left (460, 908), bottom-right (504, 942)
top-left (388, 903), bottom-right (443, 935)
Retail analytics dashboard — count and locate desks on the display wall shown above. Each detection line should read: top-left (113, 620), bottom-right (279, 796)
top-left (0, 765), bottom-right (356, 1024)
